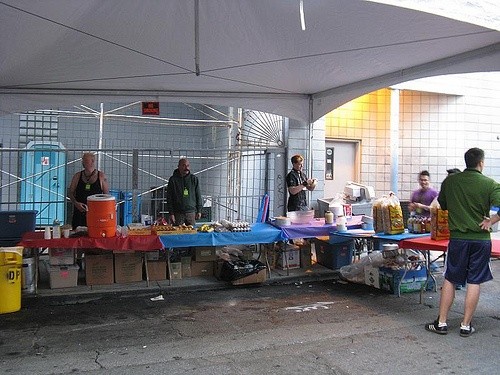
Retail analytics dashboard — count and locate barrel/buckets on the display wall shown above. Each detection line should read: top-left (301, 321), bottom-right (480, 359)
top-left (86, 194), bottom-right (117, 237)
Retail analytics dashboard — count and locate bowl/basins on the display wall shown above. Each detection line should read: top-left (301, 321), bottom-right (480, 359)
top-left (361, 223), bottom-right (374, 230)
top-left (286, 210), bottom-right (315, 226)
top-left (275, 217), bottom-right (291, 226)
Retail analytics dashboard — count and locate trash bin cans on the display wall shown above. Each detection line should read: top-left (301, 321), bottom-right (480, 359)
top-left (0, 246), bottom-right (25, 313)
top-left (22, 258), bottom-right (34, 294)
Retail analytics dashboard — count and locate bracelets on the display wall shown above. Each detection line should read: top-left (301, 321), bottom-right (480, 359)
top-left (303, 181), bottom-right (308, 186)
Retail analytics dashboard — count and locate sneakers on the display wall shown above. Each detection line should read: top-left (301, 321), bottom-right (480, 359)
top-left (425, 316), bottom-right (448, 334)
top-left (459, 322), bottom-right (476, 337)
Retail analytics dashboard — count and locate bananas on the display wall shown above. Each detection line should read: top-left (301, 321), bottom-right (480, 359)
top-left (200, 224), bottom-right (215, 232)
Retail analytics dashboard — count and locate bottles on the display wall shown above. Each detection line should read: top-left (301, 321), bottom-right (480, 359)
top-left (53, 220), bottom-right (61, 239)
top-left (336, 215), bottom-right (346, 233)
top-left (407, 215), bottom-right (432, 234)
top-left (325, 211), bottom-right (333, 224)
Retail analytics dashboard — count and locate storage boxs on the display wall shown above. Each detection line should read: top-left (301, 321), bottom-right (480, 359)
top-left (430, 207), bottom-right (450, 240)
top-left (0, 210), bottom-right (38, 248)
top-left (315, 241), bottom-right (353, 270)
top-left (43, 246), bottom-right (267, 289)
top-left (364, 265), bottom-right (379, 290)
top-left (379, 267), bottom-right (427, 295)
top-left (267, 243), bottom-right (300, 271)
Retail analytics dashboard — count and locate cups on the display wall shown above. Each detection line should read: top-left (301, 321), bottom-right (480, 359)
top-left (44, 226), bottom-right (51, 239)
top-left (63, 229), bottom-right (69, 238)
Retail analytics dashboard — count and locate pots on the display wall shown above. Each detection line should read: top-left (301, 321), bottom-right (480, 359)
top-left (382, 242), bottom-right (400, 259)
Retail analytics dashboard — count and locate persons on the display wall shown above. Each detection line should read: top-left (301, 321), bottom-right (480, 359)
top-left (425, 147), bottom-right (500, 336)
top-left (286, 155), bottom-right (316, 212)
top-left (409, 168), bottom-right (466, 290)
top-left (167, 158), bottom-right (203, 255)
top-left (68, 153), bottom-right (109, 230)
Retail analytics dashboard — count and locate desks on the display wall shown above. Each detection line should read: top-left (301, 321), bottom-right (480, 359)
top-left (330, 229), bottom-right (500, 304)
top-left (160, 223), bottom-right (286, 286)
top-left (17, 231), bottom-right (163, 297)
top-left (267, 215), bottom-right (365, 276)
